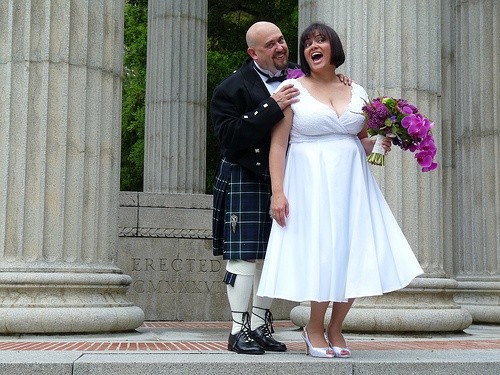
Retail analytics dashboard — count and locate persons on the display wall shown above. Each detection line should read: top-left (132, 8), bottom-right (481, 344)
top-left (255, 22), bottom-right (425, 358)
top-left (210, 21), bottom-right (354, 354)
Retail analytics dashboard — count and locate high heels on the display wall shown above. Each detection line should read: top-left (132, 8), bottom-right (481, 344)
top-left (324, 327), bottom-right (351, 357)
top-left (301, 325), bottom-right (335, 357)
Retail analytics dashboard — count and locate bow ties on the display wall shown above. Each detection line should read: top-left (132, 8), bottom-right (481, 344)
top-left (253, 62), bottom-right (287, 83)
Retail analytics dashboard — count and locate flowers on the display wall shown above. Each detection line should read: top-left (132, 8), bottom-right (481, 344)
top-left (285, 68), bottom-right (304, 79)
top-left (350, 89), bottom-right (440, 172)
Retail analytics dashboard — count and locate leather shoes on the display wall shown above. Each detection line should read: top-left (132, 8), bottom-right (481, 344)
top-left (250, 324), bottom-right (287, 352)
top-left (228, 329), bottom-right (265, 355)
